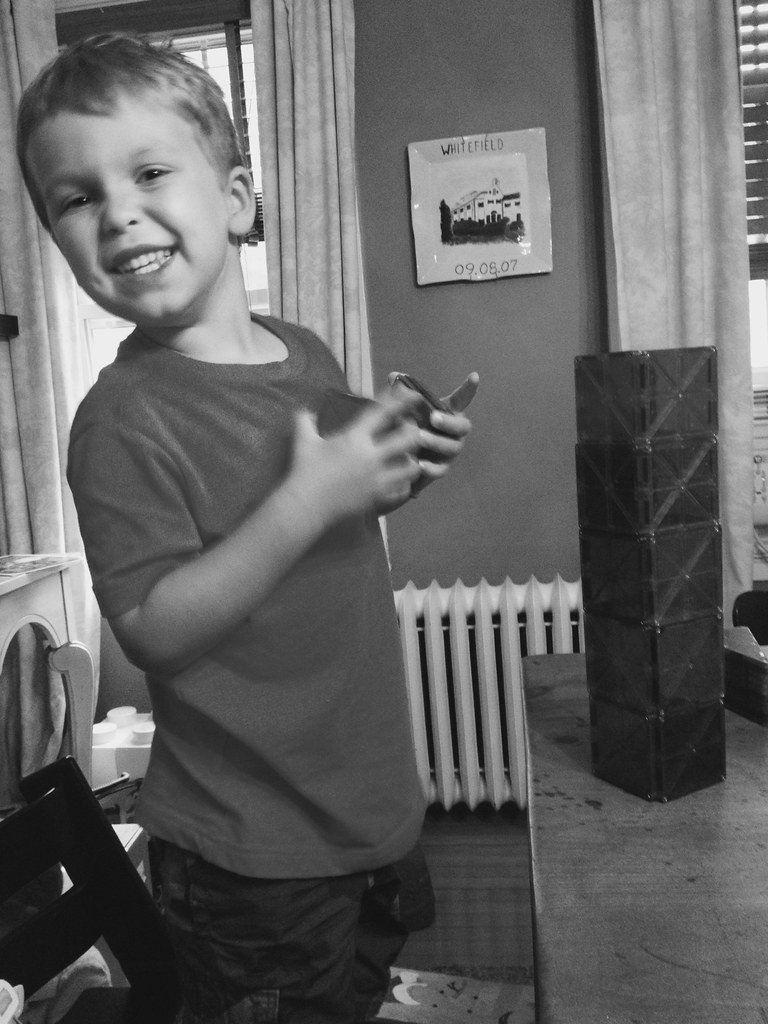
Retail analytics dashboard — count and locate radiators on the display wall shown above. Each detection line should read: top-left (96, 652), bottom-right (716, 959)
top-left (393, 572), bottom-right (580, 812)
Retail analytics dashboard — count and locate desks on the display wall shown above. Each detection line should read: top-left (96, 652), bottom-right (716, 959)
top-left (518, 651), bottom-right (768, 1024)
top-left (0, 552), bottom-right (94, 790)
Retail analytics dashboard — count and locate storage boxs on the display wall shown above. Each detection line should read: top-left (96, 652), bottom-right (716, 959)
top-left (578, 524), bottom-right (723, 624)
top-left (574, 435), bottom-right (718, 537)
top-left (588, 696), bottom-right (731, 802)
top-left (582, 613), bottom-right (726, 712)
top-left (571, 345), bottom-right (720, 444)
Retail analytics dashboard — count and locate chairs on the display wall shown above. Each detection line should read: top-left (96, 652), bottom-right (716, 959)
top-left (0, 754), bottom-right (179, 1024)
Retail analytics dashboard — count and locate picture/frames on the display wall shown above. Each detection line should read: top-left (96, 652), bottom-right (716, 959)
top-left (406, 126), bottom-right (553, 288)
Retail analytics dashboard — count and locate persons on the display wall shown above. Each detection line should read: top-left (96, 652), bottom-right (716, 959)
top-left (18, 35), bottom-right (481, 1024)
